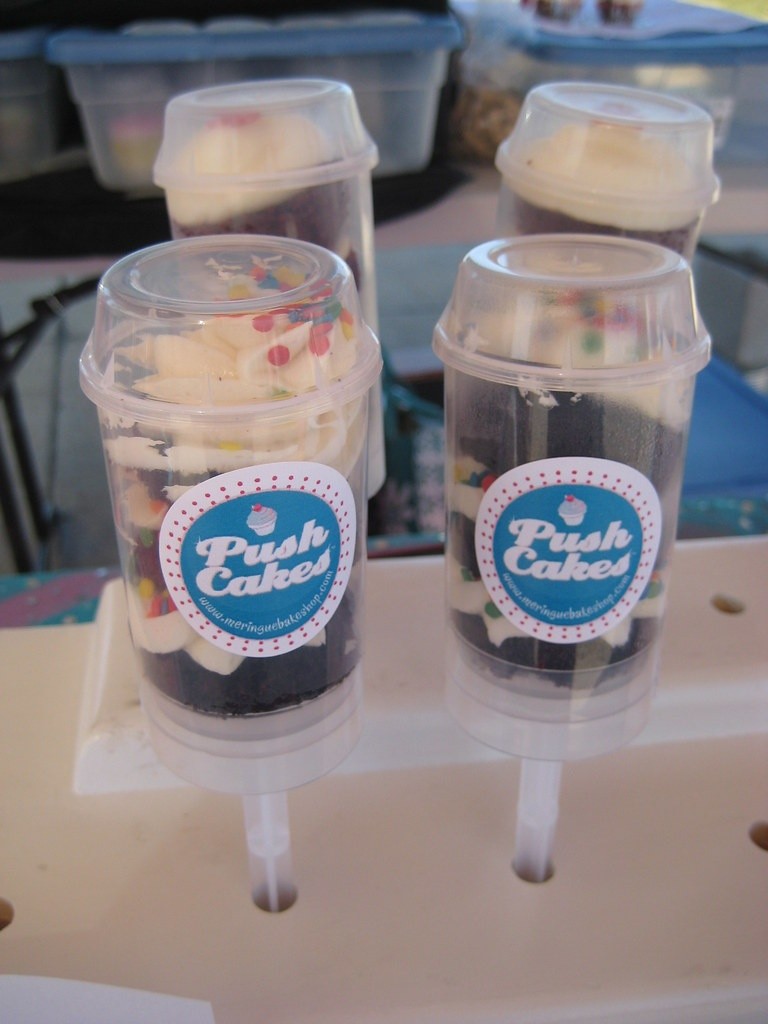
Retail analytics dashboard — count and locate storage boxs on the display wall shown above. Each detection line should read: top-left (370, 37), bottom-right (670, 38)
top-left (46, 7), bottom-right (462, 193)
top-left (1, 27), bottom-right (67, 182)
top-left (452, 1), bottom-right (767, 162)
top-left (683, 352), bottom-right (768, 535)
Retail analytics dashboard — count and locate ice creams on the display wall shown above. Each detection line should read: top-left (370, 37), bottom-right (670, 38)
top-left (157, 78), bottom-right (379, 324)
top-left (444, 264), bottom-right (691, 696)
top-left (90, 258), bottom-right (373, 715)
top-left (496, 81), bottom-right (714, 276)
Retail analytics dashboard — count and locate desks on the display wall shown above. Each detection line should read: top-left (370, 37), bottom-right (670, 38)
top-left (0, 147), bottom-right (480, 575)
